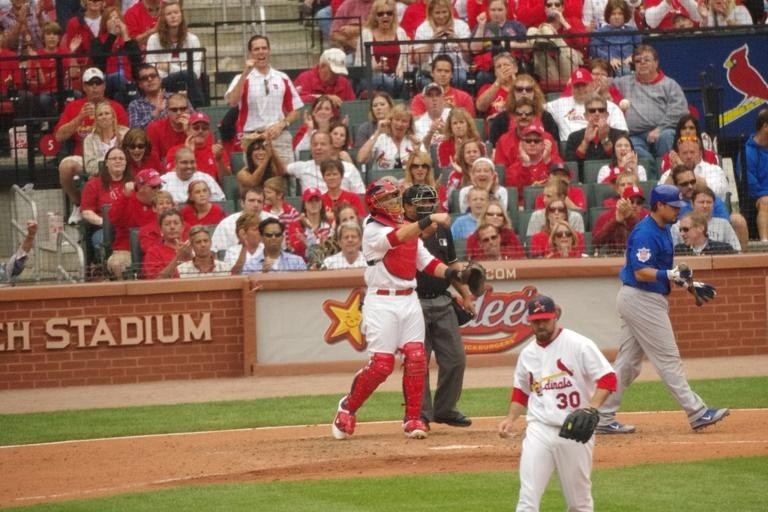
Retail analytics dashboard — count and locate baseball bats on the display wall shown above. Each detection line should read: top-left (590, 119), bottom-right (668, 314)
top-left (678, 263), bottom-right (703, 307)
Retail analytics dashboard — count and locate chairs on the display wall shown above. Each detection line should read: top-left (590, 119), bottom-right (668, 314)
top-left (91, 102), bottom-right (659, 279)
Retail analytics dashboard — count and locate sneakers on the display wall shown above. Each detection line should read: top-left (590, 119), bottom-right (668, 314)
top-left (690, 407), bottom-right (730, 433)
top-left (401, 419), bottom-right (429, 439)
top-left (332, 394), bottom-right (356, 439)
top-left (432, 416), bottom-right (472, 426)
top-left (595, 422), bottom-right (635, 434)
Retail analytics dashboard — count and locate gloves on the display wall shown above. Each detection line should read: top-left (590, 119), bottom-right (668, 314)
top-left (682, 281), bottom-right (717, 307)
top-left (666, 264), bottom-right (693, 282)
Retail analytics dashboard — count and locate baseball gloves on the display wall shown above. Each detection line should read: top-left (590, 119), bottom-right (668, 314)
top-left (559, 404), bottom-right (598, 442)
top-left (450, 264), bottom-right (486, 297)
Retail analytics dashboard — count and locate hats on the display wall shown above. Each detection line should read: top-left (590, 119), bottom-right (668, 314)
top-left (526, 296), bottom-right (557, 322)
top-left (241, 0), bottom-right (477, 162)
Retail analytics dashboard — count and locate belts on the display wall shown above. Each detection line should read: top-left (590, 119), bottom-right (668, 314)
top-left (418, 289), bottom-right (443, 299)
top-left (376, 287), bottom-right (414, 295)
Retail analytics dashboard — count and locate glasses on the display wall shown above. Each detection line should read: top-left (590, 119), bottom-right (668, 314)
top-left (479, 1), bottom-right (712, 165)
top-left (134, 163), bottom-right (516, 239)
top-left (1, 1), bottom-right (211, 166)
top-left (523, 165), bottom-right (720, 261)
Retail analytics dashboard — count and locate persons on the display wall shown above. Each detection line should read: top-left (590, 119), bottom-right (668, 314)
top-left (592, 183), bottom-right (730, 434)
top-left (329, 178), bottom-right (488, 442)
top-left (1, 0), bottom-right (767, 284)
top-left (500, 293), bottom-right (621, 512)
top-left (404, 183), bottom-right (478, 431)
